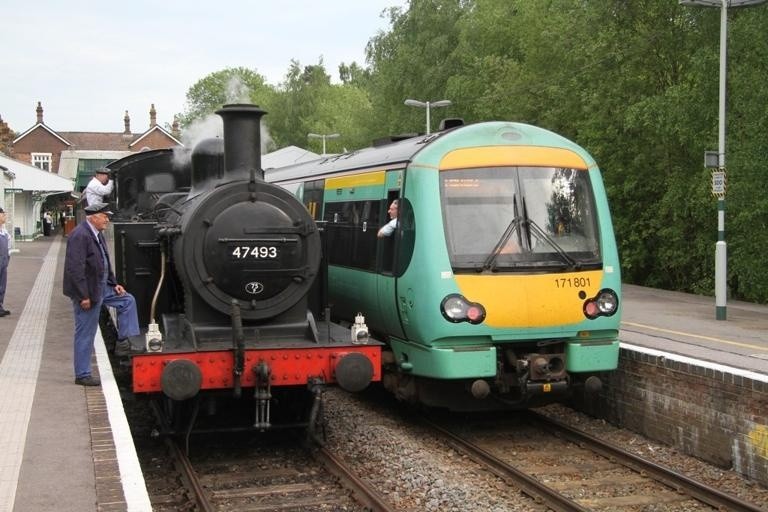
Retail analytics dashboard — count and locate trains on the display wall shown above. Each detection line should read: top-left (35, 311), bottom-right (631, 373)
top-left (106, 103), bottom-right (386, 454)
top-left (260, 121), bottom-right (621, 410)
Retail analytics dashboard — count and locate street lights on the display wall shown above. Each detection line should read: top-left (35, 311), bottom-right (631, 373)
top-left (307, 132), bottom-right (340, 154)
top-left (404, 100), bottom-right (453, 135)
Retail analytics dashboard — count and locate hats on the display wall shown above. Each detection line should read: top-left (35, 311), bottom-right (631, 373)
top-left (84, 203), bottom-right (114, 216)
top-left (96, 167), bottom-right (111, 174)
top-left (0, 208), bottom-right (7, 213)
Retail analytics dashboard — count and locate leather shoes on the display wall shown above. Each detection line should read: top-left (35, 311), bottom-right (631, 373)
top-left (115, 339), bottom-right (145, 358)
top-left (75, 376), bottom-right (100, 386)
top-left (2, 310), bottom-right (11, 317)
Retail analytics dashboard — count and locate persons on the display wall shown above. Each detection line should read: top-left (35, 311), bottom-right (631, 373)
top-left (0, 207), bottom-right (10, 317)
top-left (86, 168), bottom-right (114, 206)
top-left (63, 203), bottom-right (145, 386)
top-left (376, 199), bottom-right (399, 238)
top-left (44, 209), bottom-right (65, 236)
